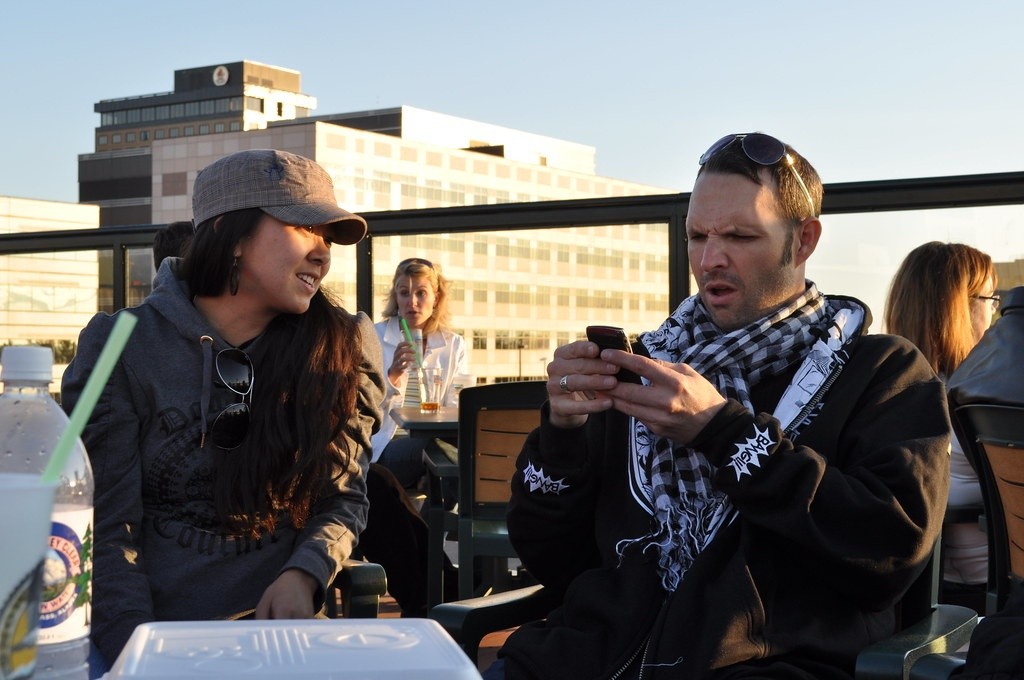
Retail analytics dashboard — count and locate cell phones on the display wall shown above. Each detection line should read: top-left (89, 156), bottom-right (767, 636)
top-left (587, 326), bottom-right (644, 386)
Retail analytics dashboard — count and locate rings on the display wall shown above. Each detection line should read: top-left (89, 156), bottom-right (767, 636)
top-left (559, 376), bottom-right (573, 394)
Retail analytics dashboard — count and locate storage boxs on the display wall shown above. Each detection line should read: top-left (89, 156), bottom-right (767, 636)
top-left (97, 619), bottom-right (484, 680)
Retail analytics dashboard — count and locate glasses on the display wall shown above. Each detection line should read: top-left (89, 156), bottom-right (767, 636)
top-left (971, 294), bottom-right (1001, 309)
top-left (698, 132), bottom-right (816, 217)
top-left (211, 346), bottom-right (254, 448)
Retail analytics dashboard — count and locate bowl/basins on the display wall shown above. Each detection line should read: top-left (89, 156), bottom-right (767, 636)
top-left (99, 619), bottom-right (484, 680)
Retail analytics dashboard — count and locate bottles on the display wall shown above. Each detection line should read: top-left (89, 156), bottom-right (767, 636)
top-left (0, 346), bottom-right (94, 680)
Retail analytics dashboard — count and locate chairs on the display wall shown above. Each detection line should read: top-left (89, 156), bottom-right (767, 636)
top-left (946, 393), bottom-right (1024, 616)
top-left (425, 537), bottom-right (978, 680)
top-left (443, 381), bottom-right (548, 602)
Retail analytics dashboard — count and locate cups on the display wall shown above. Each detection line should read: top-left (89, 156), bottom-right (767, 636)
top-left (417, 367), bottom-right (444, 414)
top-left (1, 471), bottom-right (56, 680)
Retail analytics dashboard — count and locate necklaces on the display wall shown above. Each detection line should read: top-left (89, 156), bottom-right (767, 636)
top-left (193, 295), bottom-right (197, 302)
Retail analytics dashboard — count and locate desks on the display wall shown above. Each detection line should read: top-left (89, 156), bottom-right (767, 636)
top-left (389, 407), bottom-right (458, 447)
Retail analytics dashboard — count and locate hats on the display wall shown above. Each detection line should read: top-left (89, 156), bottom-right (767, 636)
top-left (192, 148), bottom-right (367, 245)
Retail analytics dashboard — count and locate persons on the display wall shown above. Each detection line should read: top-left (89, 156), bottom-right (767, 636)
top-left (946, 285), bottom-right (1024, 408)
top-left (366, 258), bottom-right (473, 532)
top-left (55, 151), bottom-right (389, 680)
top-left (152, 222), bottom-right (493, 619)
top-left (483, 133), bottom-right (950, 679)
top-left (883, 238), bottom-right (999, 616)
